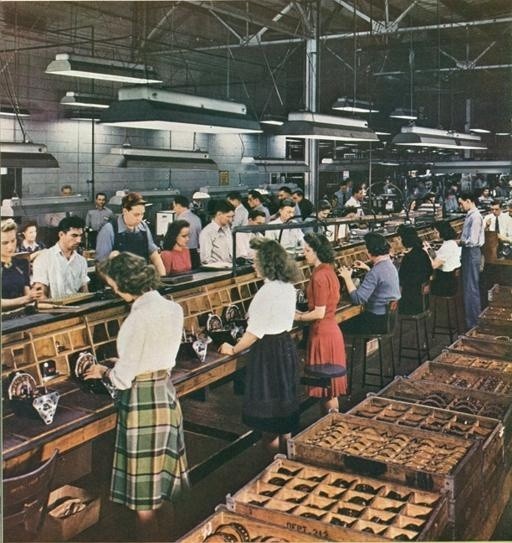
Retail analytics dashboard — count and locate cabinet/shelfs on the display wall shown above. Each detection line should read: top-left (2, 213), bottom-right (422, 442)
top-left (0, 208), bottom-right (508, 485)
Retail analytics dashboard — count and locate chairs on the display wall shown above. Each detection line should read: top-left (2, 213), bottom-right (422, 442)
top-left (397, 280), bottom-right (431, 367)
top-left (430, 266), bottom-right (464, 344)
top-left (2, 449), bottom-right (60, 542)
top-left (346, 299), bottom-right (400, 397)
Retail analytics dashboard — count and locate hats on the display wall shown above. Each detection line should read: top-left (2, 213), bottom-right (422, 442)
top-left (122, 192), bottom-right (147, 208)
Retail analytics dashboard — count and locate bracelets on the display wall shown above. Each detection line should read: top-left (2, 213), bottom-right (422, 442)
top-left (299, 312), bottom-right (302, 321)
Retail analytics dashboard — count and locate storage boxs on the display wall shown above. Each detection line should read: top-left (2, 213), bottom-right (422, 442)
top-left (345, 392), bottom-right (510, 540)
top-left (173, 505), bottom-right (335, 542)
top-left (404, 360), bottom-right (512, 399)
top-left (432, 348), bottom-right (512, 375)
top-left (376, 375), bottom-right (512, 507)
top-left (478, 307), bottom-right (512, 328)
top-left (287, 408), bottom-right (486, 542)
top-left (443, 335), bottom-right (512, 361)
top-left (463, 324), bottom-right (512, 344)
top-left (24, 483), bottom-right (100, 542)
top-left (488, 284), bottom-right (511, 308)
top-left (227, 453), bottom-right (449, 542)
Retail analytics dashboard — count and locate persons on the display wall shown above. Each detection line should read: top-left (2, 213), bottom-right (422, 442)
top-left (303, 198), bottom-right (331, 236)
top-left (15, 221), bottom-right (47, 255)
top-left (482, 200), bottom-right (512, 248)
top-left (96, 192), bottom-right (167, 278)
top-left (278, 185), bottom-right (303, 224)
top-left (236, 211), bottom-right (265, 259)
top-left (0, 218), bottom-right (43, 317)
top-left (216, 239), bottom-right (301, 462)
top-left (198, 199), bottom-right (235, 269)
top-left (247, 189), bottom-right (270, 221)
top-left (335, 167), bottom-right (512, 217)
top-left (456, 190), bottom-right (486, 331)
top-left (396, 223), bottom-right (434, 315)
top-left (423, 220), bottom-right (463, 296)
top-left (32, 214), bottom-right (91, 305)
top-left (172, 195), bottom-right (202, 271)
top-left (336, 232), bottom-right (401, 342)
top-left (82, 251), bottom-right (192, 543)
top-left (159, 220), bottom-right (191, 275)
top-left (225, 191), bottom-right (248, 231)
top-left (265, 199), bottom-right (305, 251)
top-left (293, 233), bottom-right (348, 409)
top-left (292, 190), bottom-right (314, 220)
top-left (43, 185), bottom-right (73, 248)
top-left (86, 192), bottom-right (115, 250)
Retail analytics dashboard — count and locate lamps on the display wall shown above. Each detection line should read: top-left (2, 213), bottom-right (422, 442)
top-left (59, 92), bottom-right (115, 110)
top-left (389, 108), bottom-right (417, 120)
top-left (319, 158), bottom-right (369, 172)
top-left (241, 157), bottom-right (311, 173)
top-left (274, 109), bottom-right (380, 142)
top-left (259, 115), bottom-right (287, 126)
top-left (331, 98), bottom-right (380, 113)
top-left (45, 54), bottom-right (163, 84)
top-left (193, 184), bottom-right (297, 199)
top-left (108, 189), bottom-right (180, 206)
top-left (99, 148), bottom-right (218, 172)
top-left (0, 194), bottom-right (89, 217)
top-left (0, 141), bottom-right (61, 170)
top-left (96, 88), bottom-right (263, 135)
top-left (0, 106), bottom-right (28, 117)
top-left (391, 125), bottom-right (487, 149)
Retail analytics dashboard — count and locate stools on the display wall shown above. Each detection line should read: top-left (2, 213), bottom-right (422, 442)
top-left (302, 364), bottom-right (346, 417)
